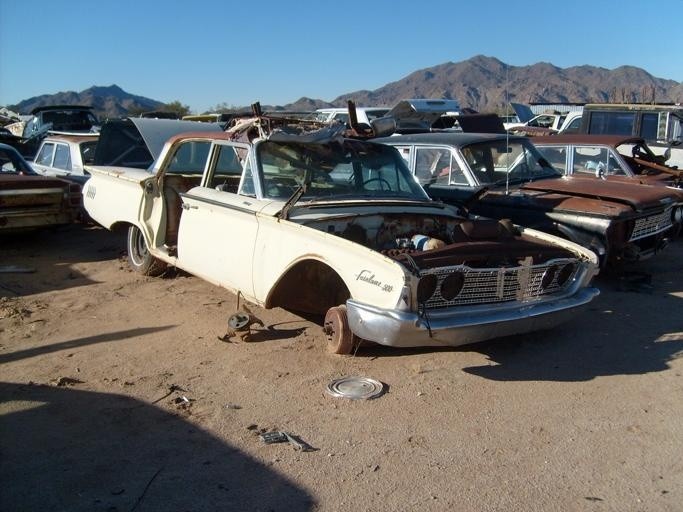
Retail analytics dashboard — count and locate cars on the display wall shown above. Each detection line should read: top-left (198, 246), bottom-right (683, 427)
top-left (99, 98), bottom-right (683, 182)
top-left (326, 131), bottom-right (683, 278)
top-left (482, 134), bottom-right (683, 190)
top-left (0, 127), bottom-right (82, 236)
top-left (2, 130), bottom-right (98, 177)
top-left (82, 131), bottom-right (602, 354)
top-left (21, 105), bottom-right (103, 140)
